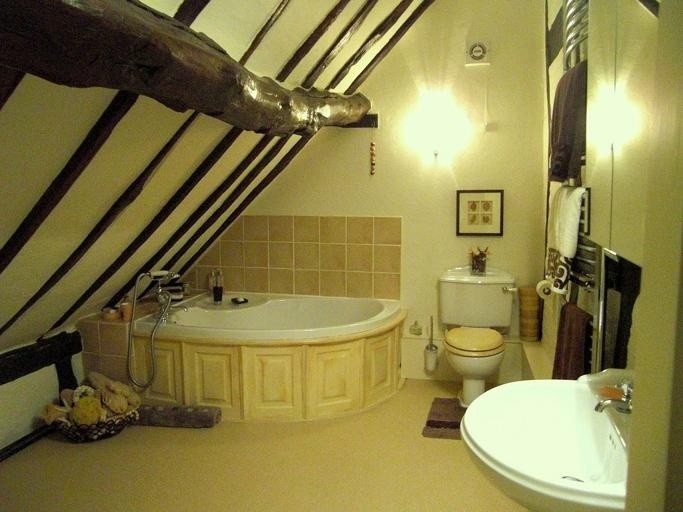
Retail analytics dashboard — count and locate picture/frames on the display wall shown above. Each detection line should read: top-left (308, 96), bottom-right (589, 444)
top-left (456, 189), bottom-right (504, 236)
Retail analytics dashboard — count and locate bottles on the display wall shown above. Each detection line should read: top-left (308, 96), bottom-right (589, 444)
top-left (207, 270), bottom-right (215, 291)
top-left (212, 268), bottom-right (223, 306)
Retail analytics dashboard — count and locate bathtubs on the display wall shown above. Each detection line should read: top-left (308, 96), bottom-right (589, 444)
top-left (135, 291), bottom-right (403, 340)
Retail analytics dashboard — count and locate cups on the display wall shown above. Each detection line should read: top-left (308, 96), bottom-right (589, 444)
top-left (101, 308), bottom-right (116, 323)
top-left (119, 301), bottom-right (131, 321)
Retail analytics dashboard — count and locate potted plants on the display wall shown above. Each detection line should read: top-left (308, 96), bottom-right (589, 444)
top-left (466, 245), bottom-right (490, 275)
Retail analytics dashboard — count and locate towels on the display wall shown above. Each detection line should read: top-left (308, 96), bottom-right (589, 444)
top-left (546, 184), bottom-right (586, 258)
top-left (552, 303), bottom-right (593, 380)
top-left (548, 59), bottom-right (587, 182)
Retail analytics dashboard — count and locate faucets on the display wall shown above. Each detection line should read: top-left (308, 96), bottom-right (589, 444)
top-left (594, 398), bottom-right (632, 415)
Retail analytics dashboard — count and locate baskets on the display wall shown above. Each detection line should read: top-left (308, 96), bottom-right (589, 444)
top-left (54, 410), bottom-right (139, 443)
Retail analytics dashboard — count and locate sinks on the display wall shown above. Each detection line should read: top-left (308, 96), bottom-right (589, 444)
top-left (460, 368), bottom-right (632, 511)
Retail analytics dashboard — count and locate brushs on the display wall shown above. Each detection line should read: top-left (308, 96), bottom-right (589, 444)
top-left (423, 317), bottom-right (438, 373)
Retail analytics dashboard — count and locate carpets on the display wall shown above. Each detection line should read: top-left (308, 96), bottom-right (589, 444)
top-left (422, 398), bottom-right (468, 440)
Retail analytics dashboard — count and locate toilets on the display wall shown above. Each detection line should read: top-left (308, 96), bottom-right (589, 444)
top-left (438, 266), bottom-right (516, 408)
